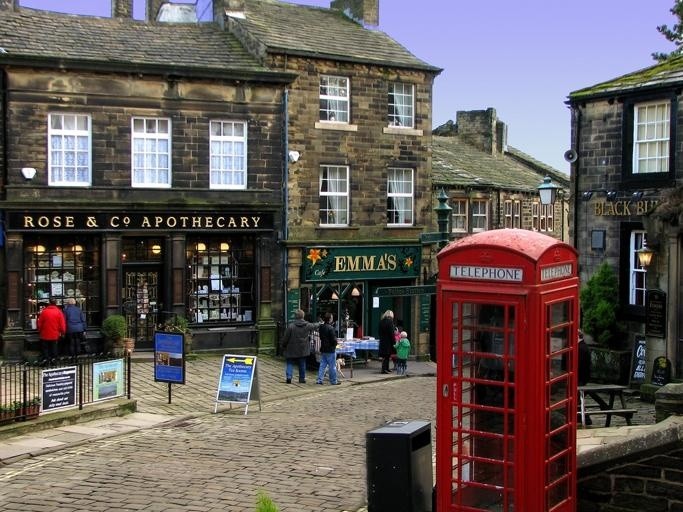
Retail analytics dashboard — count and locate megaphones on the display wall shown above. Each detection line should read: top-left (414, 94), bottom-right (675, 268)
top-left (564, 150), bottom-right (578, 163)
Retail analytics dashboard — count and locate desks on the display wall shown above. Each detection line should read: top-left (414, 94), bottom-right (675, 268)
top-left (576, 384), bottom-right (638, 426)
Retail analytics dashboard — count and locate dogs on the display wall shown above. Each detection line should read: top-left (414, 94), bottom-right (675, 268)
top-left (323, 357), bottom-right (347, 378)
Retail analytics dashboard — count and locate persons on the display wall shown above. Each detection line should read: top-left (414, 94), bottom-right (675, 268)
top-left (390, 327), bottom-right (400, 370)
top-left (577, 329), bottom-right (591, 426)
top-left (377, 309), bottom-right (394, 375)
top-left (62, 298), bottom-right (87, 354)
top-left (36, 295), bottom-right (66, 368)
top-left (274, 308), bottom-right (324, 383)
top-left (315, 312), bottom-right (340, 385)
top-left (393, 331), bottom-right (410, 378)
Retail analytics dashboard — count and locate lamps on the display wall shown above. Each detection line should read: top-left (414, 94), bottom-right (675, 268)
top-left (578, 190), bottom-right (646, 204)
top-left (536, 174), bottom-right (575, 206)
top-left (633, 245), bottom-right (661, 271)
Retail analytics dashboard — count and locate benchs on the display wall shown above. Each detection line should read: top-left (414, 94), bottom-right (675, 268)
top-left (575, 408), bottom-right (636, 420)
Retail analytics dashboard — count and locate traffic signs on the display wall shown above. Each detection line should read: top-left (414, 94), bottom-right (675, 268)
top-left (374, 285), bottom-right (439, 296)
top-left (419, 231), bottom-right (444, 243)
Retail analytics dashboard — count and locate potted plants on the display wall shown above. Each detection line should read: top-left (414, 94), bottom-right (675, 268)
top-left (0, 397), bottom-right (41, 426)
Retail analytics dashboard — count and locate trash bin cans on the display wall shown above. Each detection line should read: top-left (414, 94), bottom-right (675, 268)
top-left (365, 417), bottom-right (433, 512)
